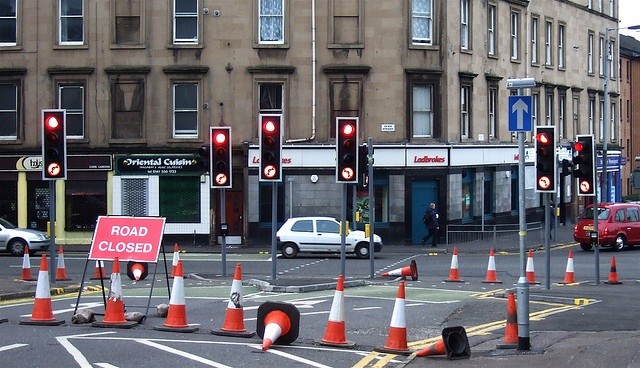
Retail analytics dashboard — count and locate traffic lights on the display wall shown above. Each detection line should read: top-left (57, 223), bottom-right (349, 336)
top-left (573, 134), bottom-right (596, 196)
top-left (336, 117), bottom-right (358, 183)
top-left (259, 114), bottom-right (282, 182)
top-left (534, 126), bottom-right (556, 193)
top-left (210, 127), bottom-right (232, 188)
top-left (359, 146), bottom-right (374, 187)
top-left (42, 110), bottom-right (67, 180)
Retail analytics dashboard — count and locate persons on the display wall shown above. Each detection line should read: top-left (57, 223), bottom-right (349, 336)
top-left (421, 203), bottom-right (440, 247)
top-left (543, 199), bottom-right (554, 240)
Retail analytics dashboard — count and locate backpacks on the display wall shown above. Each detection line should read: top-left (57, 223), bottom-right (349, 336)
top-left (423, 209), bottom-right (435, 225)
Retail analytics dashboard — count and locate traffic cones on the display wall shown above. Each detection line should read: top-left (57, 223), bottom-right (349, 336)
top-left (496, 290), bottom-right (519, 348)
top-left (319, 274), bottom-right (357, 349)
top-left (482, 244), bottom-right (503, 283)
top-left (211, 262), bottom-right (255, 338)
top-left (168, 242), bottom-right (187, 279)
top-left (374, 280), bottom-right (413, 356)
top-left (91, 256), bottom-right (138, 328)
top-left (153, 259), bottom-right (199, 333)
top-left (256, 300), bottom-right (300, 350)
top-left (90, 260), bottom-right (110, 280)
top-left (525, 249), bottom-right (541, 284)
top-left (127, 261), bottom-right (148, 281)
top-left (416, 326), bottom-right (471, 360)
top-left (381, 260), bottom-right (418, 281)
top-left (443, 245), bottom-right (465, 282)
top-left (19, 245), bottom-right (37, 281)
top-left (604, 255), bottom-right (622, 285)
top-left (55, 244), bottom-right (72, 281)
top-left (19, 253), bottom-right (65, 325)
top-left (558, 248), bottom-right (575, 284)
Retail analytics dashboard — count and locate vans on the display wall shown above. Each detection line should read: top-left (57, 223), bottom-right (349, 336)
top-left (573, 202), bottom-right (640, 251)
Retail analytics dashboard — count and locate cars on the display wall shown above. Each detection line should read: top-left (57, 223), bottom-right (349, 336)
top-left (276, 216), bottom-right (383, 259)
top-left (0, 218), bottom-right (50, 256)
